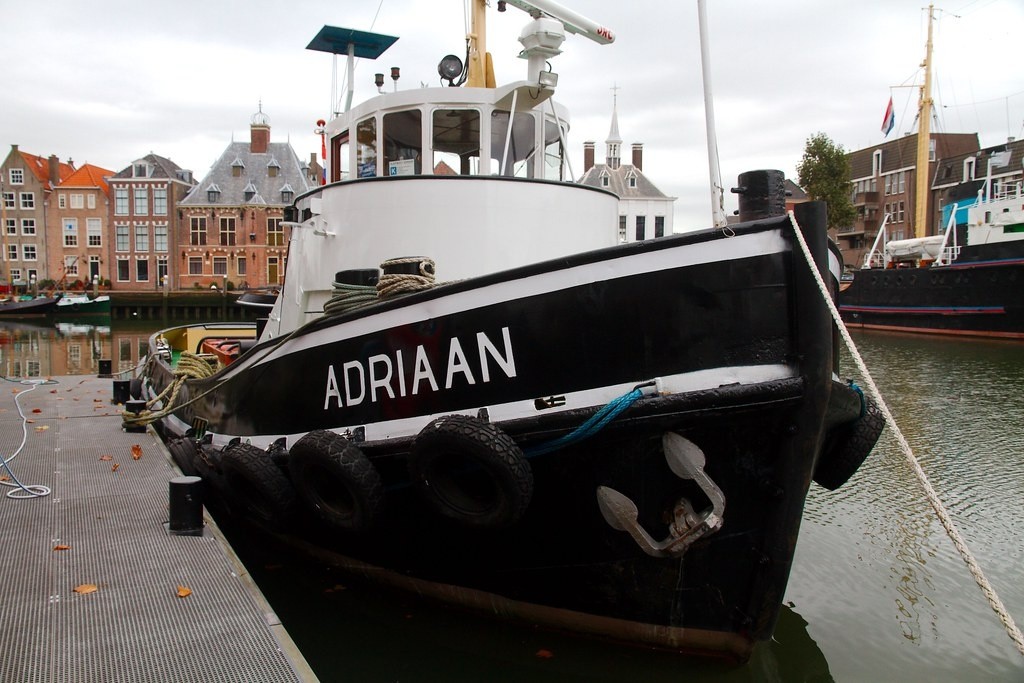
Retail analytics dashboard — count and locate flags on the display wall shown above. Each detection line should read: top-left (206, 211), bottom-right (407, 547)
top-left (881, 97), bottom-right (894, 139)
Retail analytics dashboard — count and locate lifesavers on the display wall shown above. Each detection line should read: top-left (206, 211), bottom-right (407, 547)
top-left (409, 415), bottom-right (535, 541)
top-left (169, 437), bottom-right (197, 476)
top-left (193, 449), bottom-right (222, 486)
top-left (813, 393), bottom-right (886, 491)
top-left (289, 431), bottom-right (377, 519)
top-left (222, 442), bottom-right (285, 504)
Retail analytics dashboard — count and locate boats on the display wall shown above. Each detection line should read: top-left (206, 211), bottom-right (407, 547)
top-left (234, 290), bottom-right (280, 323)
top-left (110, 0), bottom-right (886, 683)
top-left (0, 294), bottom-right (64, 323)
